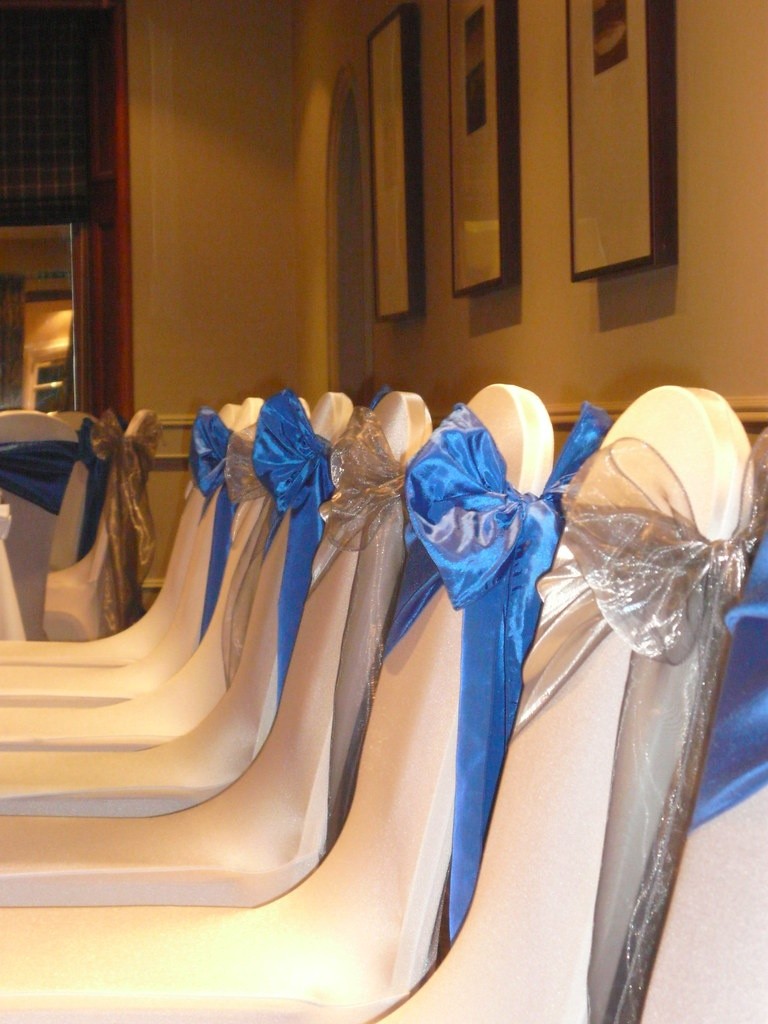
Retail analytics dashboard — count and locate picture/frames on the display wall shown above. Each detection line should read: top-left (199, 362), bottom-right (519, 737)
top-left (447, 0), bottom-right (521, 296)
top-left (566, 0), bottom-right (676, 284)
top-left (369, 2), bottom-right (426, 322)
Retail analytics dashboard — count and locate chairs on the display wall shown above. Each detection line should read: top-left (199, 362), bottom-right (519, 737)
top-left (0, 385), bottom-right (768, 1024)
